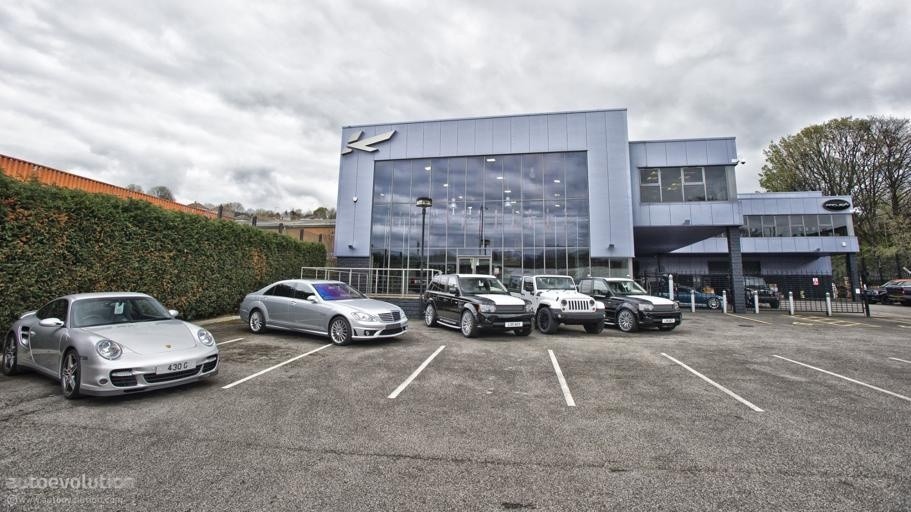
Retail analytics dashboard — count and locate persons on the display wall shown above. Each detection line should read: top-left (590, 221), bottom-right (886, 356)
top-left (743, 223), bottom-right (828, 236)
top-left (770, 284), bottom-right (778, 293)
top-left (701, 285), bottom-right (715, 294)
top-left (831, 281), bottom-right (838, 302)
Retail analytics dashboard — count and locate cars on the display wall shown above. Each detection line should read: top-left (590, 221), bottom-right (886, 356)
top-left (887, 279), bottom-right (911, 304)
top-left (237, 278), bottom-right (410, 345)
top-left (652, 284), bottom-right (724, 310)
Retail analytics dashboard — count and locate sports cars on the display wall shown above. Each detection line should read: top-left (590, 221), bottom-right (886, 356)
top-left (1, 291), bottom-right (219, 398)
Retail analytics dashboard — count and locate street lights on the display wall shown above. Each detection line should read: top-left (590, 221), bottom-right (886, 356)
top-left (481, 239), bottom-right (491, 256)
top-left (414, 196), bottom-right (432, 319)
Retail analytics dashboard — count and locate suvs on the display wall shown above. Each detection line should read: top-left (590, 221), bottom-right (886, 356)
top-left (726, 274), bottom-right (780, 309)
top-left (407, 270), bottom-right (445, 292)
top-left (506, 273), bottom-right (605, 335)
top-left (860, 279), bottom-right (904, 305)
top-left (422, 273), bottom-right (529, 337)
top-left (574, 276), bottom-right (680, 332)
